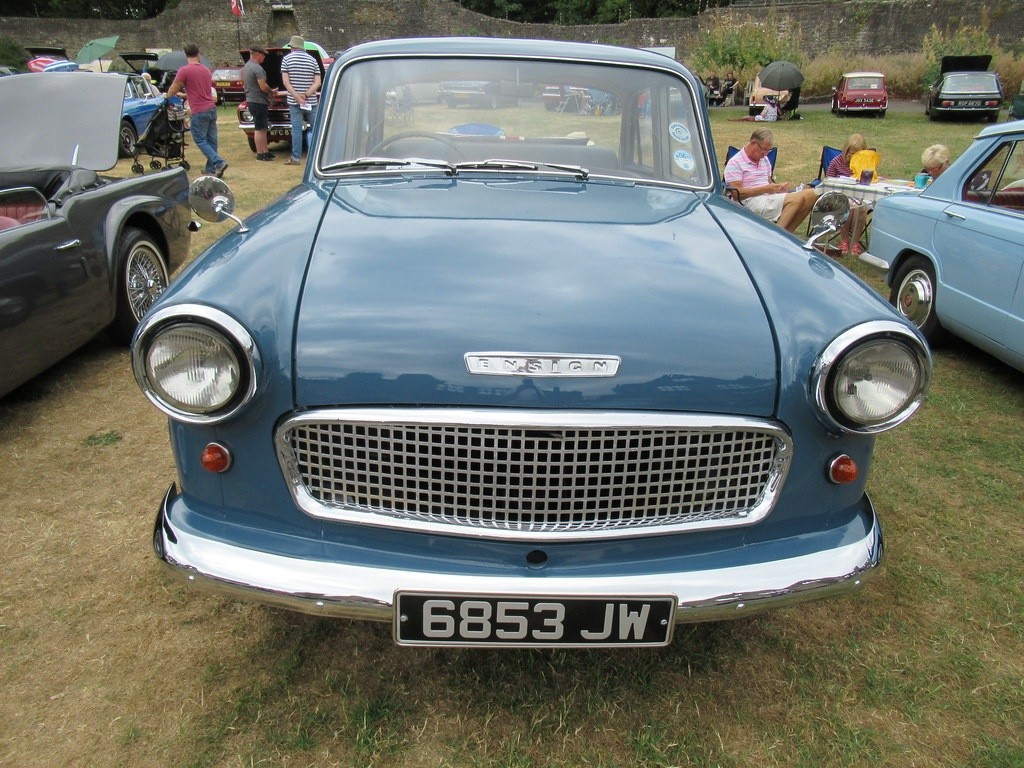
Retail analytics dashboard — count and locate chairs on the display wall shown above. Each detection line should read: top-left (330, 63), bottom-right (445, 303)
top-left (720, 146), bottom-right (778, 209)
top-left (779, 87), bottom-right (800, 121)
top-left (557, 85), bottom-right (581, 114)
top-left (390, 84), bottom-right (416, 126)
top-left (743, 80), bottom-right (755, 105)
top-left (806, 145), bottom-right (878, 251)
top-left (720, 81), bottom-right (739, 106)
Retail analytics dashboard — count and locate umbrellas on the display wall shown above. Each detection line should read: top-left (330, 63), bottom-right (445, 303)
top-left (282, 41), bottom-right (329, 60)
top-left (153, 49), bottom-right (211, 70)
top-left (28, 57), bottom-right (79, 72)
top-left (757, 61), bottom-right (804, 91)
top-left (75, 36), bottom-right (120, 71)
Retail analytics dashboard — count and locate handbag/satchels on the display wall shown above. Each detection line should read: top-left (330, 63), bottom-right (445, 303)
top-left (725, 98), bottom-right (731, 107)
top-left (849, 150), bottom-right (880, 183)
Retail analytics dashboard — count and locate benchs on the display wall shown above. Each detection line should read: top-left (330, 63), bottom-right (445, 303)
top-left (0, 203), bottom-right (44, 223)
top-left (387, 139), bottom-right (620, 171)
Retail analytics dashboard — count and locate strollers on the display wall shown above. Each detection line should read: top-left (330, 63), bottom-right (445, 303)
top-left (131, 94), bottom-right (191, 174)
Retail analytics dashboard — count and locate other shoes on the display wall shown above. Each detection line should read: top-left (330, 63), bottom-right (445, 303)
top-left (201, 170), bottom-right (215, 176)
top-left (719, 102), bottom-right (724, 107)
top-left (257, 153), bottom-right (276, 162)
top-left (839, 241), bottom-right (850, 256)
top-left (214, 161), bottom-right (228, 178)
top-left (851, 243), bottom-right (861, 257)
top-left (282, 158), bottom-right (301, 165)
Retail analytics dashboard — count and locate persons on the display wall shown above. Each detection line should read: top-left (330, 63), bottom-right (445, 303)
top-left (914, 145), bottom-right (950, 182)
top-left (281, 36), bottom-right (322, 165)
top-left (162, 43), bottom-right (228, 178)
top-left (717, 73), bottom-right (738, 107)
top-left (243, 45), bottom-right (279, 161)
top-left (781, 86), bottom-right (801, 114)
top-left (706, 72), bottom-right (719, 105)
top-left (825, 134), bottom-right (868, 254)
top-left (137, 73), bottom-right (160, 98)
top-left (724, 127), bottom-right (817, 233)
top-left (166, 71), bottom-right (184, 94)
top-left (743, 95), bottom-right (780, 122)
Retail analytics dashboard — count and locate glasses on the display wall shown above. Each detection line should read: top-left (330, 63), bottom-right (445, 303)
top-left (756, 142), bottom-right (773, 152)
top-left (929, 163), bottom-right (943, 177)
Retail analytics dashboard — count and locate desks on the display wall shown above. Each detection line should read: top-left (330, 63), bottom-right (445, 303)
top-left (815, 176), bottom-right (928, 256)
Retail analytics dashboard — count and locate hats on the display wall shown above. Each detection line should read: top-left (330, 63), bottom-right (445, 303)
top-left (249, 44), bottom-right (269, 55)
top-left (764, 95), bottom-right (776, 108)
top-left (288, 36), bottom-right (306, 52)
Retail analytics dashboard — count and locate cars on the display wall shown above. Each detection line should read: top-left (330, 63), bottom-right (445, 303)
top-left (441, 79), bottom-right (522, 110)
top-left (831, 72), bottom-right (889, 118)
top-left (925, 55), bottom-right (1005, 123)
top-left (105, 72), bottom-right (184, 159)
top-left (129, 36), bottom-right (937, 649)
top-left (237, 48), bottom-right (337, 152)
top-left (0, 70), bottom-right (203, 401)
top-left (857, 119), bottom-right (1024, 377)
top-left (211, 66), bottom-right (246, 106)
